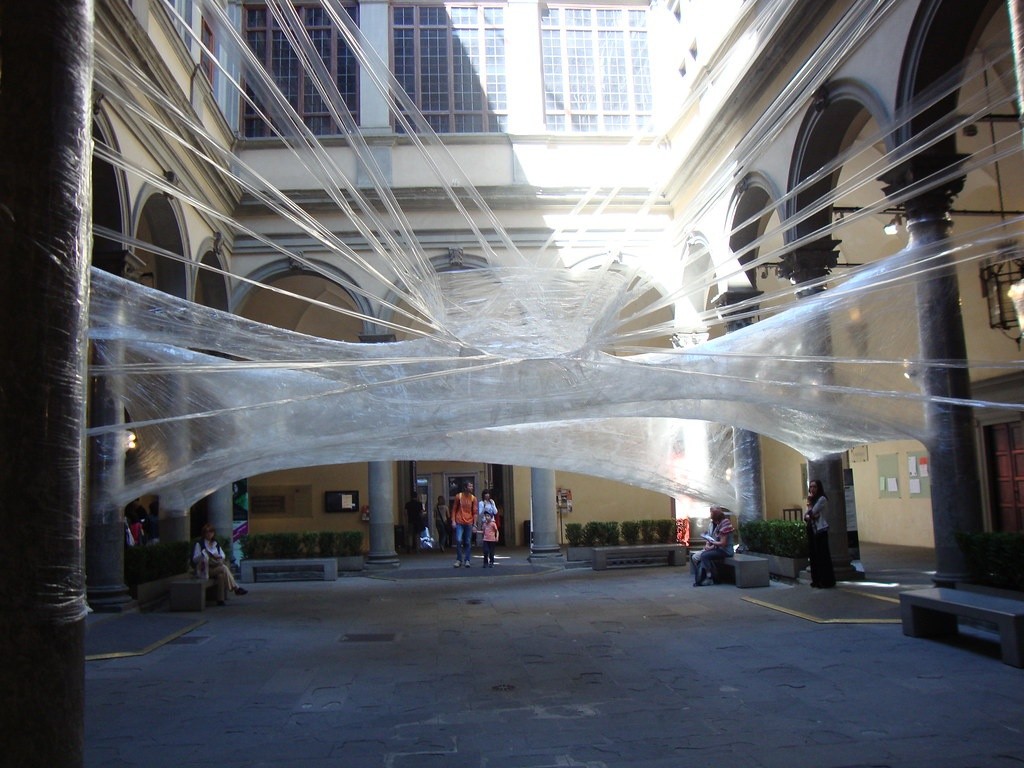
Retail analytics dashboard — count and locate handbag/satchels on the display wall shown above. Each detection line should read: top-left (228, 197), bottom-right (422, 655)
top-left (209, 556), bottom-right (223, 568)
top-left (496, 513), bottom-right (501, 528)
top-left (445, 523), bottom-right (452, 534)
top-left (197, 549), bottom-right (209, 580)
top-left (414, 514), bottom-right (424, 532)
top-left (692, 549), bottom-right (707, 566)
top-left (124, 517), bottom-right (134, 547)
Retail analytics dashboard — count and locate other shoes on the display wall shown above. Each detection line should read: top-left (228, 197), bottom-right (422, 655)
top-left (235, 588), bottom-right (248, 595)
top-left (465, 560), bottom-right (470, 568)
top-left (454, 560), bottom-right (461, 568)
top-left (216, 600), bottom-right (225, 606)
top-left (701, 578), bottom-right (714, 586)
top-left (489, 561), bottom-right (494, 568)
top-left (483, 562), bottom-right (488, 568)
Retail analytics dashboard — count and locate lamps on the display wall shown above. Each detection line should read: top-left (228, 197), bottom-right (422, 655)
top-left (883, 219), bottom-right (897, 235)
top-left (977, 53), bottom-right (1024, 351)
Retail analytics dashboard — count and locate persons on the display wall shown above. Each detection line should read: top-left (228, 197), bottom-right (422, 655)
top-left (452, 480), bottom-right (500, 569)
top-left (803, 480), bottom-right (836, 588)
top-left (692, 506), bottom-right (733, 587)
top-left (433, 495), bottom-right (450, 553)
top-left (404, 491), bottom-right (424, 555)
top-left (194, 525), bottom-right (248, 607)
top-left (124, 497), bottom-right (159, 547)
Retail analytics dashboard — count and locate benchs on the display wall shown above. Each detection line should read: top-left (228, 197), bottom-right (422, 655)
top-left (590, 543), bottom-right (687, 570)
top-left (171, 575), bottom-right (217, 612)
top-left (899, 587), bottom-right (1023, 667)
top-left (688, 550), bottom-right (769, 588)
top-left (241, 558), bottom-right (338, 583)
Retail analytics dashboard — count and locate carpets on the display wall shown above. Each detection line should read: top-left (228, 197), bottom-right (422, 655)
top-left (740, 586), bottom-right (901, 624)
top-left (83, 613), bottom-right (207, 661)
top-left (372, 563), bottom-right (563, 582)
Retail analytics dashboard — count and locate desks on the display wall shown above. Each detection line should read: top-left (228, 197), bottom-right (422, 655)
top-left (782, 509), bottom-right (802, 521)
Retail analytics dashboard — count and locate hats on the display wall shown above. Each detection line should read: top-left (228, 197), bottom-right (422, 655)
top-left (484, 509), bottom-right (493, 517)
